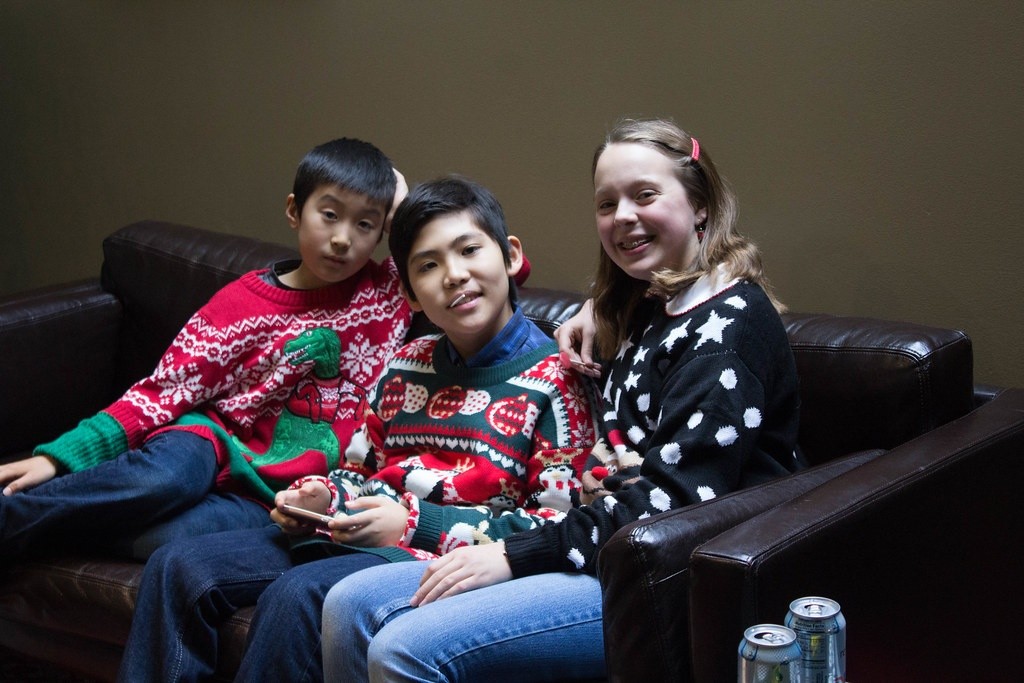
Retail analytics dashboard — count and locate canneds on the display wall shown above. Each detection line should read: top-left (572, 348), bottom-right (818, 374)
top-left (736, 624), bottom-right (804, 683)
top-left (784, 596), bottom-right (847, 683)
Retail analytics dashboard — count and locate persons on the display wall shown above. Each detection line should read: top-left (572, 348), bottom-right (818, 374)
top-left (320, 112), bottom-right (804, 683)
top-left (116, 169), bottom-right (602, 682)
top-left (0, 137), bottom-right (531, 570)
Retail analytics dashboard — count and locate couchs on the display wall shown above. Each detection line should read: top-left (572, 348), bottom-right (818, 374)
top-left (0, 218), bottom-right (1024, 683)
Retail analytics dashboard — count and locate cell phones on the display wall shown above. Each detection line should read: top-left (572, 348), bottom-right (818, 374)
top-left (279, 503), bottom-right (357, 533)
